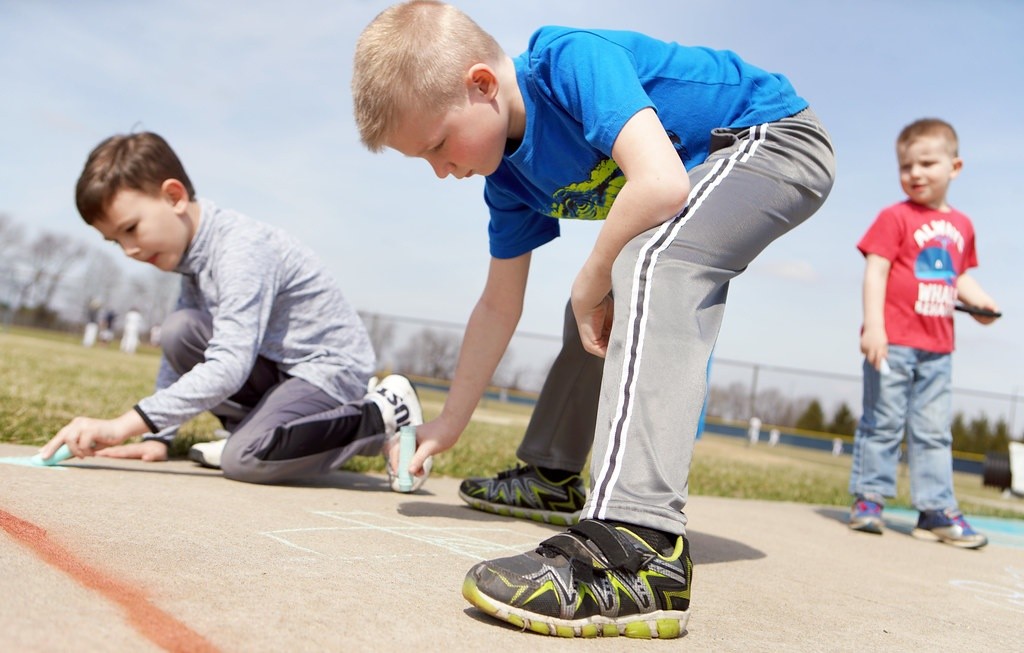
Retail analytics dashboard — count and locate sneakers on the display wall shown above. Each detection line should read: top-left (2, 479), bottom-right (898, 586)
top-left (462, 518), bottom-right (693, 638)
top-left (848, 492), bottom-right (886, 533)
top-left (189, 430), bottom-right (232, 466)
top-left (364, 374), bottom-right (433, 491)
top-left (912, 508), bottom-right (988, 547)
top-left (459, 462), bottom-right (586, 526)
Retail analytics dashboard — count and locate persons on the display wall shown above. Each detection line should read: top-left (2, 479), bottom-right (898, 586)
top-left (849, 117), bottom-right (1000, 548)
top-left (82, 299), bottom-right (164, 354)
top-left (353, 0), bottom-right (838, 639)
top-left (41, 129), bottom-right (433, 495)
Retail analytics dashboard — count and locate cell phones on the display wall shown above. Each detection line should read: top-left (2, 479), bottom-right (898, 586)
top-left (955, 305), bottom-right (1001, 317)
